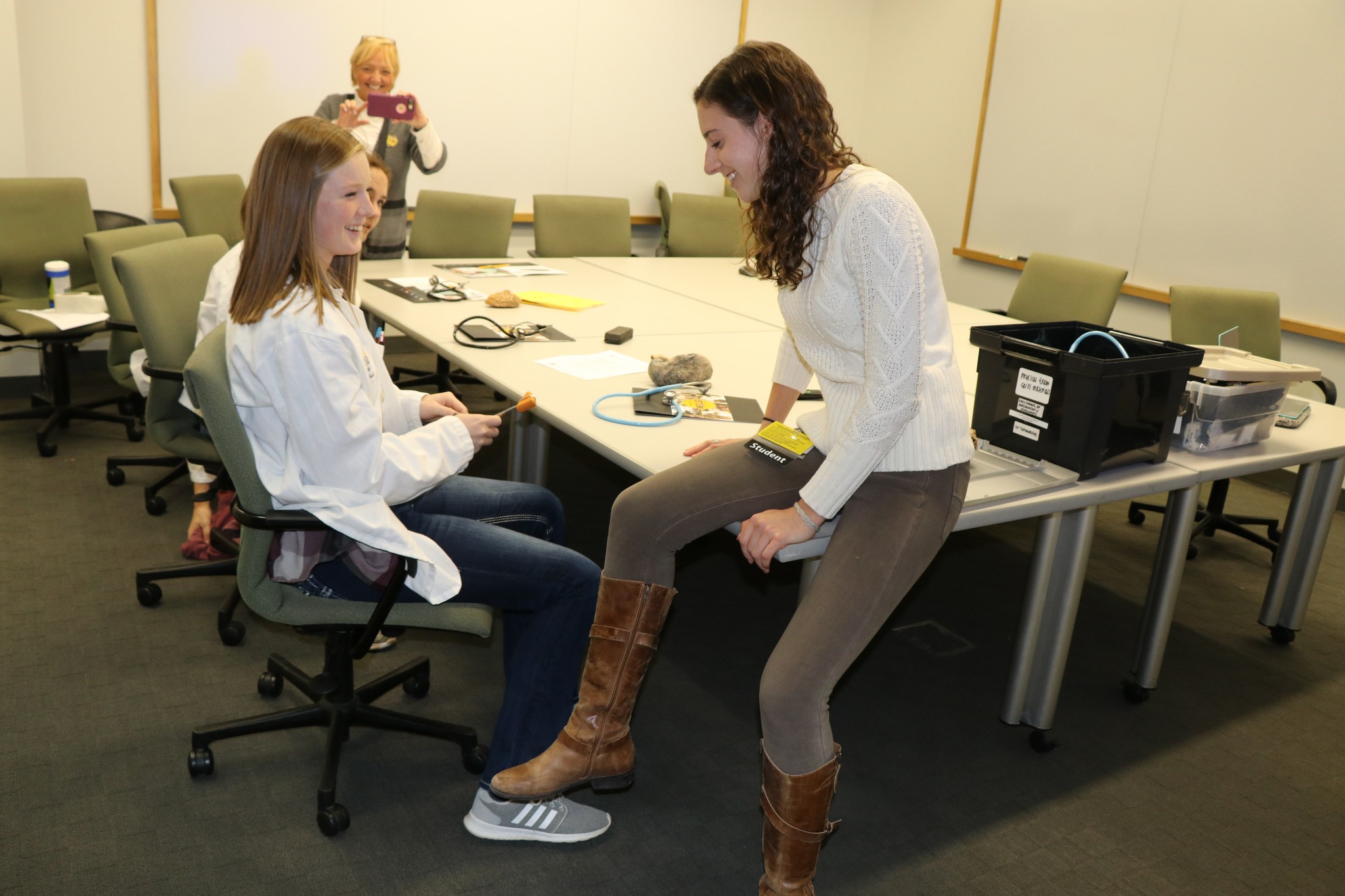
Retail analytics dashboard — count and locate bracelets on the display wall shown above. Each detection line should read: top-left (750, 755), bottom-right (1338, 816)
top-left (793, 501), bottom-right (821, 532)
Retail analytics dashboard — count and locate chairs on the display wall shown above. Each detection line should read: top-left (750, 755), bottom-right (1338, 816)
top-left (0, 174), bottom-right (776, 837)
top-left (982, 252), bottom-right (1128, 328)
top-left (1127, 286), bottom-right (1337, 566)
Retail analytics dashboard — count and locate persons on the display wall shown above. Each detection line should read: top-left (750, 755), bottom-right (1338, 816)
top-left (490, 39), bottom-right (973, 896)
top-left (188, 114), bottom-right (612, 841)
top-left (315, 36), bottom-right (446, 259)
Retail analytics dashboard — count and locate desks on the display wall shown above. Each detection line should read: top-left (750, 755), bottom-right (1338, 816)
top-left (586, 257), bottom-right (1345, 713)
top-left (362, 256), bottom-right (1200, 785)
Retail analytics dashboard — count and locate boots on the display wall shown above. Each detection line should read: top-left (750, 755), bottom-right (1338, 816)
top-left (754, 737), bottom-right (841, 896)
top-left (488, 569), bottom-right (678, 799)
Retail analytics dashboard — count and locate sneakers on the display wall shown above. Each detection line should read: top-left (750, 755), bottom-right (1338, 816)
top-left (462, 787), bottom-right (612, 842)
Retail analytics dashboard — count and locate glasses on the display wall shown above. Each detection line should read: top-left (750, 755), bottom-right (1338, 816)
top-left (360, 36), bottom-right (396, 47)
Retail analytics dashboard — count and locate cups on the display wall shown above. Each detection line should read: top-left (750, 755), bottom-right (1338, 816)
top-left (44, 260), bottom-right (71, 308)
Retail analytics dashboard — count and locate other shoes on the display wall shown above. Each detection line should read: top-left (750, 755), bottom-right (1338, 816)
top-left (371, 630), bottom-right (398, 649)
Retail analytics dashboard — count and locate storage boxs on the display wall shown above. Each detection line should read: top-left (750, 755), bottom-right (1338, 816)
top-left (1172, 344), bottom-right (1322, 453)
top-left (970, 320), bottom-right (1205, 482)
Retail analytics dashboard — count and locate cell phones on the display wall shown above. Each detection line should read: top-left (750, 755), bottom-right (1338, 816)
top-left (798, 389), bottom-right (822, 400)
top-left (367, 93), bottom-right (415, 120)
